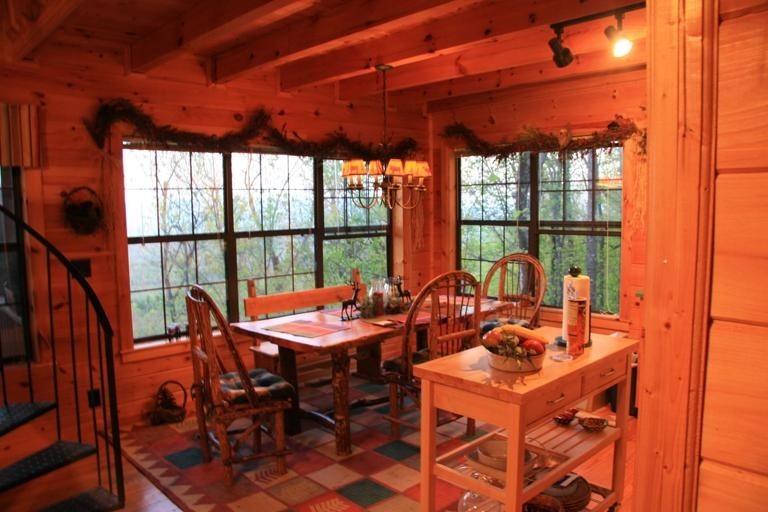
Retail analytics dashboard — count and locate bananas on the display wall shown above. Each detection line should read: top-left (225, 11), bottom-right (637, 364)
top-left (502, 324), bottom-right (548, 344)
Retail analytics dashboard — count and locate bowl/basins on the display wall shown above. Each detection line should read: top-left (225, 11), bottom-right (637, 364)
top-left (486, 346), bottom-right (546, 375)
top-left (553, 408), bottom-right (609, 431)
top-left (476, 438), bottom-right (530, 469)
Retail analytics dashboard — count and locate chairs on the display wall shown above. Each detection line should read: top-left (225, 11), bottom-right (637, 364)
top-left (381, 253), bottom-right (548, 441)
top-left (181, 286), bottom-right (297, 485)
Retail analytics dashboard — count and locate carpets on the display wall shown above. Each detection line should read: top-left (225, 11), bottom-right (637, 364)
top-left (92, 370), bottom-right (499, 512)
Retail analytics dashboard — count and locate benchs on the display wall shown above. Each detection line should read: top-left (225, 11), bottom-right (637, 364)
top-left (242, 266), bottom-right (380, 387)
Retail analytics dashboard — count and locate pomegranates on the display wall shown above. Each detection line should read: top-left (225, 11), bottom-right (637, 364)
top-left (482, 329), bottom-right (502, 347)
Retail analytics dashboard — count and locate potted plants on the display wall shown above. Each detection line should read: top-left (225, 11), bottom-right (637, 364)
top-left (61, 183), bottom-right (107, 237)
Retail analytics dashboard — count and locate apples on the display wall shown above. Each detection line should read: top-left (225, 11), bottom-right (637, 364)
top-left (515, 346), bottom-right (527, 356)
top-left (522, 339), bottom-right (545, 354)
top-left (508, 334), bottom-right (519, 347)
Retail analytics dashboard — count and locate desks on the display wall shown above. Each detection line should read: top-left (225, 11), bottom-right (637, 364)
top-left (414, 335), bottom-right (639, 512)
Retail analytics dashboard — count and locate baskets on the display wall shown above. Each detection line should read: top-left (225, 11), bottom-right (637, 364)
top-left (150, 380), bottom-right (188, 426)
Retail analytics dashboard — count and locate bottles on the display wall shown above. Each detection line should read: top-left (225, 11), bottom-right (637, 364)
top-left (368, 275), bottom-right (411, 313)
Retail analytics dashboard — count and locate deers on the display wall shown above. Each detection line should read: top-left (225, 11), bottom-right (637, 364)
top-left (340, 279), bottom-right (363, 321)
top-left (394, 274), bottom-right (412, 304)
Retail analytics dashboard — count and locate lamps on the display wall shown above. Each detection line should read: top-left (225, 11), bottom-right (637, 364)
top-left (545, 1), bottom-right (647, 70)
top-left (336, 62), bottom-right (440, 213)
